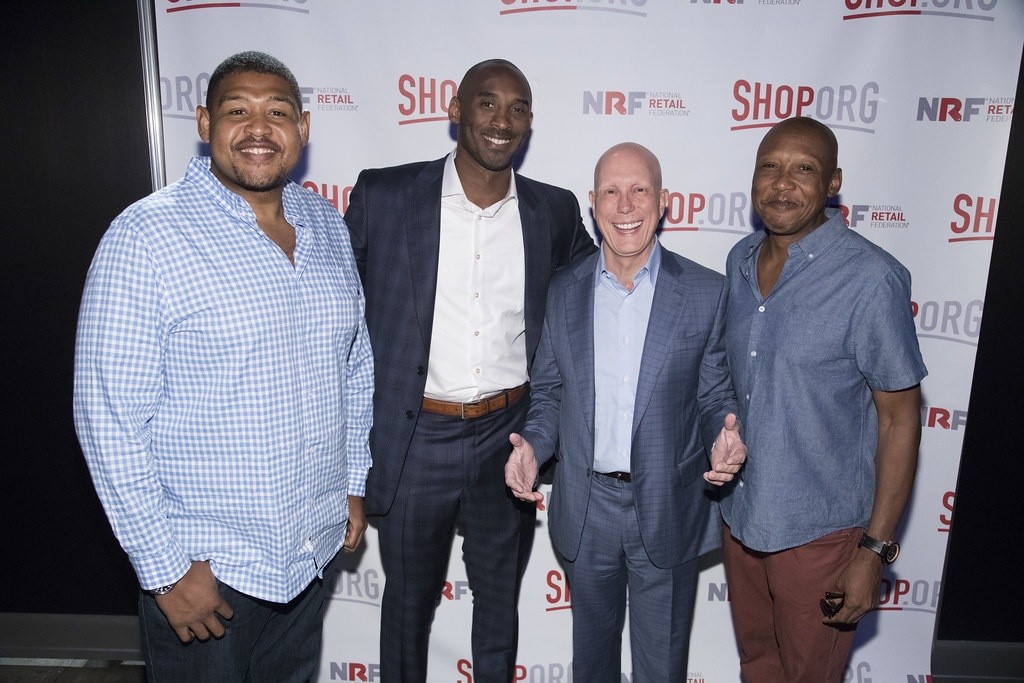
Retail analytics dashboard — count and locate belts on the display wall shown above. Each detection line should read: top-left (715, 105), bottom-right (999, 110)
top-left (420, 383), bottom-right (529, 420)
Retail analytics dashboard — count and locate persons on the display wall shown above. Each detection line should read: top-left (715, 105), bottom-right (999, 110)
top-left (328, 57), bottom-right (597, 682)
top-left (504, 128), bottom-right (747, 682)
top-left (723, 114), bottom-right (931, 683)
top-left (71, 47), bottom-right (373, 680)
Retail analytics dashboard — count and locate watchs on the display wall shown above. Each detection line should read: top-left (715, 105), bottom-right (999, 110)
top-left (150, 583), bottom-right (175, 596)
top-left (856, 531), bottom-right (901, 566)
